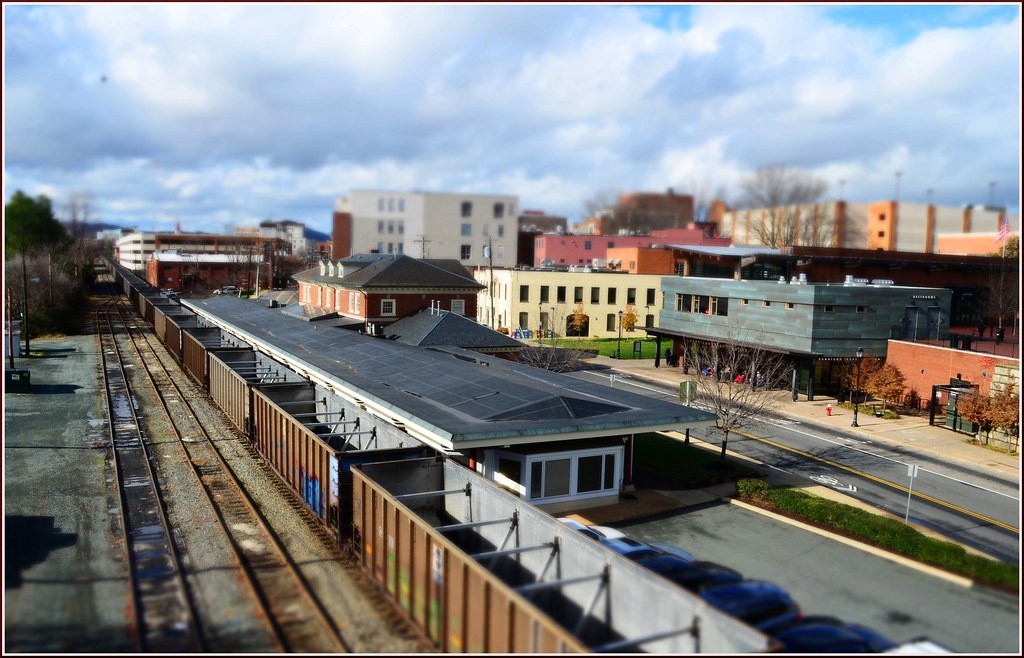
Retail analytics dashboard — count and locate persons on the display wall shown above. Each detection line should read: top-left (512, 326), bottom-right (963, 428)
top-left (664, 348), bottom-right (671, 365)
top-left (703, 359), bottom-right (769, 392)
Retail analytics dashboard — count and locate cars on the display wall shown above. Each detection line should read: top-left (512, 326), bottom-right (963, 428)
top-left (560, 517), bottom-right (896, 653)
top-left (213, 286), bottom-right (240, 295)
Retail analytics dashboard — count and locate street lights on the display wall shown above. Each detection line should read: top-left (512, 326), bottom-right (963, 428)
top-left (539, 302), bottom-right (541, 345)
top-left (21, 279), bottom-right (40, 341)
top-left (852, 346), bottom-right (864, 428)
top-left (617, 311), bottom-right (622, 359)
top-left (550, 308), bottom-right (554, 342)
top-left (645, 306), bottom-right (650, 339)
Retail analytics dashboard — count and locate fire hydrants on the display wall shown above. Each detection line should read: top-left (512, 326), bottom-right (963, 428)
top-left (826, 404), bottom-right (832, 416)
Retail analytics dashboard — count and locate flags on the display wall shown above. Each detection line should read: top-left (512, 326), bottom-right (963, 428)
top-left (995, 216), bottom-right (1011, 244)
